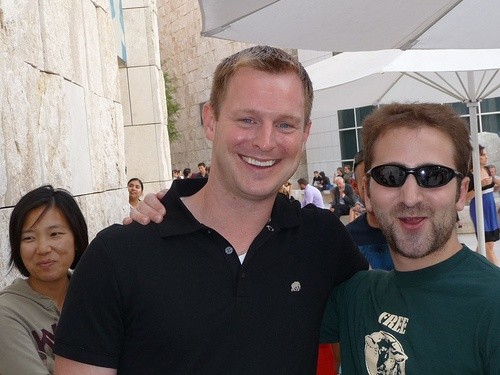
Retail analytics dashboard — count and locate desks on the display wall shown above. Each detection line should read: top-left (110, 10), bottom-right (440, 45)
top-left (290, 190), bottom-right (306, 203)
top-left (349, 196), bottom-right (476, 235)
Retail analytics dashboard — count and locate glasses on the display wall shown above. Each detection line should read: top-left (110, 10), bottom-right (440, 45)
top-left (364, 165), bottom-right (465, 189)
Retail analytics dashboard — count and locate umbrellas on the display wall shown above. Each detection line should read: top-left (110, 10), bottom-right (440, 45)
top-left (304, 49), bottom-right (500, 258)
top-left (198, 0), bottom-right (500, 51)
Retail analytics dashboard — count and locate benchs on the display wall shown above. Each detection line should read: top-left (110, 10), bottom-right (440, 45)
top-left (319, 189), bottom-right (333, 203)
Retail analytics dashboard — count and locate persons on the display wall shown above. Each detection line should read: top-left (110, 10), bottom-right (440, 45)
top-left (51, 45), bottom-right (370, 375)
top-left (127, 143), bottom-right (499, 272)
top-left (122, 100), bottom-right (500, 375)
top-left (0, 185), bottom-right (89, 375)
